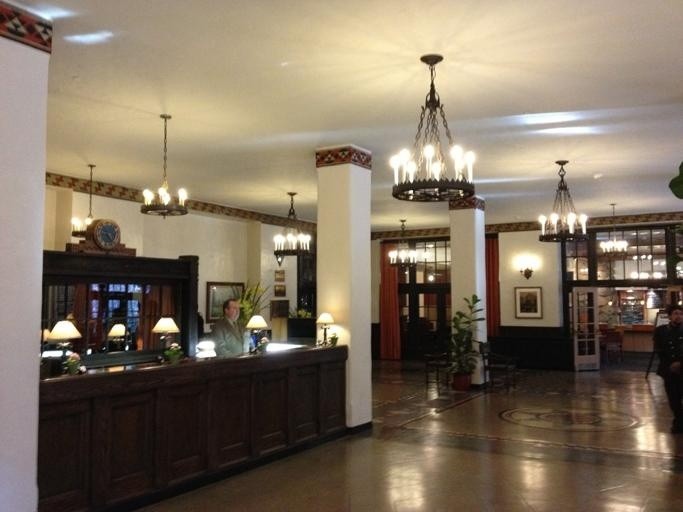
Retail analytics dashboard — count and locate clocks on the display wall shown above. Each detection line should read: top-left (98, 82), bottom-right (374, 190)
top-left (67, 218), bottom-right (136, 257)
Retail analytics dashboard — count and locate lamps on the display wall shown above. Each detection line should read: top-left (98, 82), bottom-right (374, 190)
top-left (46, 313), bottom-right (334, 372)
top-left (599, 204), bottom-right (629, 256)
top-left (387, 219), bottom-right (420, 267)
top-left (518, 266), bottom-right (532, 279)
top-left (140, 113), bottom-right (189, 216)
top-left (538, 160), bottom-right (590, 241)
top-left (70, 163), bottom-right (96, 237)
top-left (273, 191), bottom-right (311, 255)
top-left (387, 54), bottom-right (475, 202)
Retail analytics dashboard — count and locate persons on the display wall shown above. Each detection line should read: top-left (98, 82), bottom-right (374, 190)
top-left (654, 306), bottom-right (683, 433)
top-left (211, 298), bottom-right (243, 358)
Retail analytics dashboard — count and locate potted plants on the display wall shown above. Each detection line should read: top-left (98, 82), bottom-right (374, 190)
top-left (441, 294), bottom-right (487, 390)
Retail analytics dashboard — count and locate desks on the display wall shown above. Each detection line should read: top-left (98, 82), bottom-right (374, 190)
top-left (620, 325), bottom-right (655, 353)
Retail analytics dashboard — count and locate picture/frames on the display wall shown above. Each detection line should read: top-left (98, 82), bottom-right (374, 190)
top-left (207, 281), bottom-right (244, 322)
top-left (514, 287), bottom-right (543, 319)
